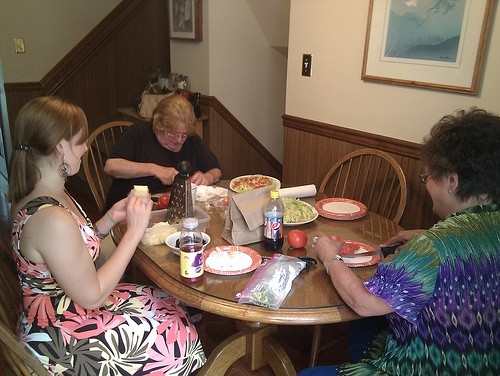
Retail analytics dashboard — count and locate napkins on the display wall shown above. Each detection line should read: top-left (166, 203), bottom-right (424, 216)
top-left (277, 184), bottom-right (317, 198)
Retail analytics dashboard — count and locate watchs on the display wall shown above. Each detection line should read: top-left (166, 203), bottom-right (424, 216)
top-left (325, 254), bottom-right (343, 273)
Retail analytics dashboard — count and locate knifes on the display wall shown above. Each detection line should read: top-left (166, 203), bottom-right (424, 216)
top-left (338, 243), bottom-right (404, 258)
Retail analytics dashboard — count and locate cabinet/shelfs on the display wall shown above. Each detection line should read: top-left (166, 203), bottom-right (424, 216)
top-left (118, 107), bottom-right (208, 140)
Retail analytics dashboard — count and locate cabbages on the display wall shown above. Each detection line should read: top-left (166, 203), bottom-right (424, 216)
top-left (281, 197), bottom-right (313, 222)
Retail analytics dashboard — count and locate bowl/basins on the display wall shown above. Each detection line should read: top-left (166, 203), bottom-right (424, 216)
top-left (164, 231), bottom-right (211, 255)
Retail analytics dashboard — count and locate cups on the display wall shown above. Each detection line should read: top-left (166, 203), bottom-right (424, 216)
top-left (191, 183), bottom-right (197, 211)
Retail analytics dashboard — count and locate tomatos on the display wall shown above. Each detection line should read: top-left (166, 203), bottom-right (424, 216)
top-left (339, 242), bottom-right (360, 255)
top-left (233, 176), bottom-right (270, 189)
top-left (158, 195), bottom-right (170, 208)
top-left (287, 230), bottom-right (307, 249)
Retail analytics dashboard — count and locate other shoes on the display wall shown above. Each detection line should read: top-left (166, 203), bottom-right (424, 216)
top-left (187, 306), bottom-right (203, 325)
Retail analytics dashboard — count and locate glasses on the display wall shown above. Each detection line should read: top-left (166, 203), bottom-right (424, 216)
top-left (420, 173), bottom-right (429, 184)
top-left (166, 128), bottom-right (187, 140)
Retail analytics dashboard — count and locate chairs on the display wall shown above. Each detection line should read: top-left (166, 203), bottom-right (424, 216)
top-left (83, 121), bottom-right (133, 216)
top-left (309, 148), bottom-right (407, 367)
top-left (0, 236), bottom-right (50, 376)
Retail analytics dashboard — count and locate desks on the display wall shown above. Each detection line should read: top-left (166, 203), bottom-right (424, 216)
top-left (111, 180), bottom-right (404, 376)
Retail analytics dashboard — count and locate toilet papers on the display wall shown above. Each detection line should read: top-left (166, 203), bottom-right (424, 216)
top-left (280, 182), bottom-right (317, 199)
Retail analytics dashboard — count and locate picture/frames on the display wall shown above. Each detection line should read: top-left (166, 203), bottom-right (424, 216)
top-left (361, 0), bottom-right (497, 96)
top-left (168, 0), bottom-right (203, 41)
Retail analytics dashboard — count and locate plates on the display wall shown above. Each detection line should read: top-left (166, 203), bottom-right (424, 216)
top-left (283, 200), bottom-right (318, 226)
top-left (203, 246), bottom-right (262, 276)
top-left (196, 185), bottom-right (229, 201)
top-left (229, 174), bottom-right (281, 193)
top-left (315, 198), bottom-right (368, 220)
top-left (336, 239), bottom-right (381, 268)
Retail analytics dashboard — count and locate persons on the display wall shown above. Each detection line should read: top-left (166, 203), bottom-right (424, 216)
top-left (298, 105), bottom-right (500, 376)
top-left (6, 97), bottom-right (210, 376)
top-left (102, 98), bottom-right (222, 324)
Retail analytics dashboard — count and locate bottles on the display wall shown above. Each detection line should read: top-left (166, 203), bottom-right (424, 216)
top-left (179, 218), bottom-right (204, 283)
top-left (263, 190), bottom-right (284, 252)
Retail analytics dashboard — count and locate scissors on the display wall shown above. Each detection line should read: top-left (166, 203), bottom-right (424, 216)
top-left (262, 256), bottom-right (317, 270)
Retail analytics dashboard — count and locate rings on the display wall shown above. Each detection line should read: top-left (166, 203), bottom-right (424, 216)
top-left (313, 235), bottom-right (319, 243)
